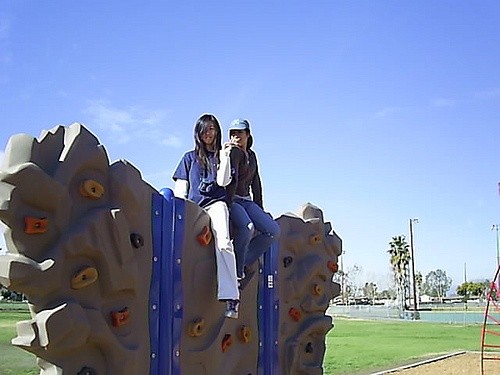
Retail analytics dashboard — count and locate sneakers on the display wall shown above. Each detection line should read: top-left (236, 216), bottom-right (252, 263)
top-left (225, 299), bottom-right (240, 319)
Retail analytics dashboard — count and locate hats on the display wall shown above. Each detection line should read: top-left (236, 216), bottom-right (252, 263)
top-left (229, 118), bottom-right (249, 130)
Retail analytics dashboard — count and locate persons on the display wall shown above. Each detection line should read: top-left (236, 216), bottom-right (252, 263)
top-left (221, 118), bottom-right (280, 291)
top-left (171, 113), bottom-right (243, 320)
